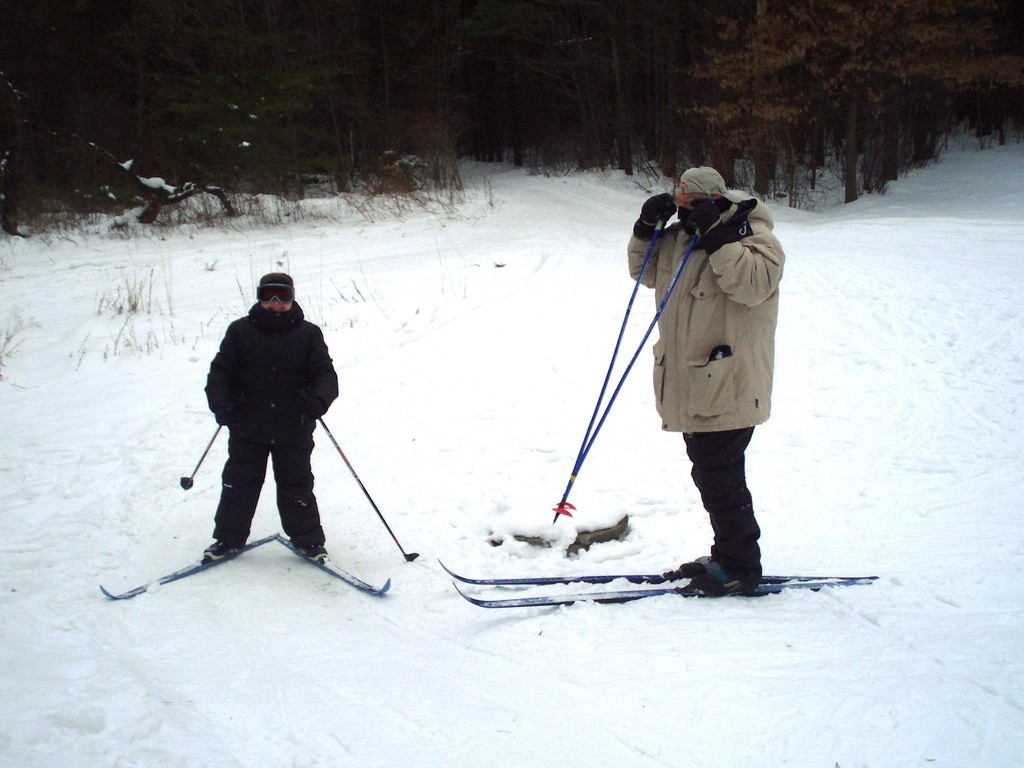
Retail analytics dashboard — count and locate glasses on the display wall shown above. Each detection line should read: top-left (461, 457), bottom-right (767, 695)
top-left (258, 284), bottom-right (296, 303)
top-left (674, 197), bottom-right (693, 208)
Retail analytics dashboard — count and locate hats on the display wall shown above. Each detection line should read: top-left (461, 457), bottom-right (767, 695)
top-left (675, 166), bottom-right (728, 195)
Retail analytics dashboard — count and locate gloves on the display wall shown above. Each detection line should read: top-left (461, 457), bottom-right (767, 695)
top-left (215, 402), bottom-right (237, 426)
top-left (300, 394), bottom-right (328, 419)
top-left (634, 193), bottom-right (677, 241)
top-left (690, 198), bottom-right (723, 240)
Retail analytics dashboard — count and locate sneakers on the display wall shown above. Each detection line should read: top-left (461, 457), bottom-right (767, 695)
top-left (692, 570), bottom-right (762, 596)
top-left (289, 537), bottom-right (327, 561)
top-left (204, 542), bottom-right (239, 560)
top-left (679, 555), bottom-right (722, 577)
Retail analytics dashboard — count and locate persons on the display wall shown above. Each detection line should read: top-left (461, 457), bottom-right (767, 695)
top-left (202, 275), bottom-right (340, 561)
top-left (628, 164), bottom-right (784, 596)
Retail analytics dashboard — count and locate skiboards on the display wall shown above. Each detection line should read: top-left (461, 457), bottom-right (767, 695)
top-left (97, 528), bottom-right (392, 608)
top-left (435, 555), bottom-right (885, 612)
